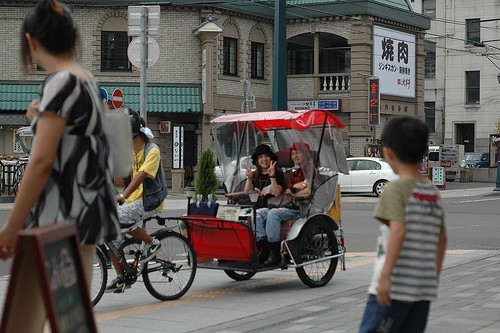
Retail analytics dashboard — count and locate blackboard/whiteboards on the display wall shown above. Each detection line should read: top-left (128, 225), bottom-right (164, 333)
top-left (0, 223), bottom-right (98, 333)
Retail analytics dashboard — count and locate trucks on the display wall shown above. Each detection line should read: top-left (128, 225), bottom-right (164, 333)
top-left (419, 145), bottom-right (459, 182)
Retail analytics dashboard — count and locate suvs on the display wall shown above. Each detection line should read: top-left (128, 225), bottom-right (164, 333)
top-left (213, 156), bottom-right (317, 195)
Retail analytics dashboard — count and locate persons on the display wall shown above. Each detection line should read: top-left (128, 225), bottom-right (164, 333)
top-left (0, 0), bottom-right (122, 333)
top-left (218, 142), bottom-right (321, 267)
top-left (355, 116), bottom-right (447, 333)
top-left (104, 117), bottom-right (168, 292)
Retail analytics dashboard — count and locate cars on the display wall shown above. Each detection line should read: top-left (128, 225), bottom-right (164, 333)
top-left (464, 152), bottom-right (490, 168)
top-left (318, 156), bottom-right (397, 196)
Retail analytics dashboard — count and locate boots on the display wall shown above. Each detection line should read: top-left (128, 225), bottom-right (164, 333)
top-left (263, 241), bottom-right (282, 267)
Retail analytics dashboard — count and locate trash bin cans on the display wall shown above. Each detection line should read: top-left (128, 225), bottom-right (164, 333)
top-left (460, 169), bottom-right (470, 183)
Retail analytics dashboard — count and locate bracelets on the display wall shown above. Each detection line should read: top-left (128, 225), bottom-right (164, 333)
top-left (268, 176), bottom-right (276, 178)
top-left (119, 194), bottom-right (125, 201)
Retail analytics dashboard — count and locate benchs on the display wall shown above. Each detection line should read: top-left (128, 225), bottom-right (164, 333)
top-left (231, 150), bottom-right (330, 239)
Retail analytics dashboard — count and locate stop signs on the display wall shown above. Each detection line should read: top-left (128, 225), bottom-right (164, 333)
top-left (112, 88), bottom-right (124, 109)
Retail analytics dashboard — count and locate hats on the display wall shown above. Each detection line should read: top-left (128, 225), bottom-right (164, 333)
top-left (289, 142), bottom-right (313, 161)
top-left (252, 144), bottom-right (277, 166)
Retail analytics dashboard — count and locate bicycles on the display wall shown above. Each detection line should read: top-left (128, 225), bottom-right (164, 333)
top-left (82, 199), bottom-right (195, 310)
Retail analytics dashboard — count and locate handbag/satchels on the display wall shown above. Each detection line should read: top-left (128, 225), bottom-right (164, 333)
top-left (266, 193), bottom-right (307, 211)
top-left (84, 72), bottom-right (132, 178)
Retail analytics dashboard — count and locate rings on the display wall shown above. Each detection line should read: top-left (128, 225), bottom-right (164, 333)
top-left (2, 248), bottom-right (8, 252)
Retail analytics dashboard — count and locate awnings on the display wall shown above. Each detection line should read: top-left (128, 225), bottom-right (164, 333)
top-left (0, 81), bottom-right (203, 112)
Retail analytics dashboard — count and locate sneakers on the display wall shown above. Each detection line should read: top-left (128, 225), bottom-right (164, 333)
top-left (98, 276), bottom-right (131, 293)
top-left (138, 237), bottom-right (161, 264)
